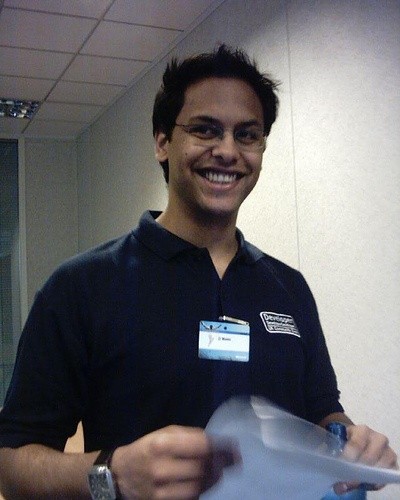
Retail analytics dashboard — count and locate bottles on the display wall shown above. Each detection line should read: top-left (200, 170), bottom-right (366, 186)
top-left (321, 422), bottom-right (368, 500)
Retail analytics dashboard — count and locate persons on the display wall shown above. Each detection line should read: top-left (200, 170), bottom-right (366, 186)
top-left (0, 44), bottom-right (400, 500)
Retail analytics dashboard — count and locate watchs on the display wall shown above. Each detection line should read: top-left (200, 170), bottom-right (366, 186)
top-left (86, 448), bottom-right (119, 500)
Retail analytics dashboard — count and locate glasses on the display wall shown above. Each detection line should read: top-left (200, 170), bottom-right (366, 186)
top-left (174, 123), bottom-right (269, 153)
